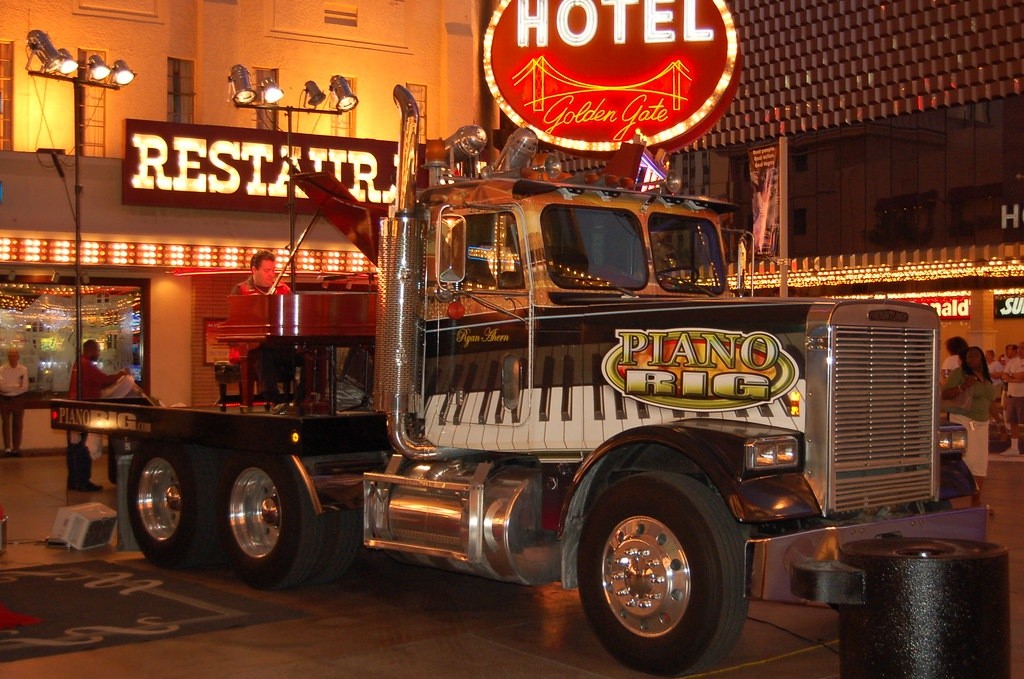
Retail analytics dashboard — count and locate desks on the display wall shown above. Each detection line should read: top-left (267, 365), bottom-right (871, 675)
top-left (247, 333), bottom-right (373, 413)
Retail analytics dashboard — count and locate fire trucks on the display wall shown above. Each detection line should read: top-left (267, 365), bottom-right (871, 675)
top-left (48, 85), bottom-right (989, 679)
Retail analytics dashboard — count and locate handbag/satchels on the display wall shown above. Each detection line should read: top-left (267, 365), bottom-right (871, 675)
top-left (943, 385), bottom-right (974, 409)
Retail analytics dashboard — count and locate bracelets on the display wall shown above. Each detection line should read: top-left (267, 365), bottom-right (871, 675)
top-left (958, 384), bottom-right (964, 393)
top-left (1010, 373), bottom-right (1011, 376)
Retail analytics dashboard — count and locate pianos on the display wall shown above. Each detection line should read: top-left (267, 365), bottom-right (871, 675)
top-left (213, 274), bottom-right (381, 415)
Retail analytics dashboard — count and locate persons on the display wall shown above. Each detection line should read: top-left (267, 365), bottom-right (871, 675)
top-left (66, 339), bottom-right (131, 492)
top-left (228, 251), bottom-right (321, 404)
top-left (938, 337), bottom-right (1024, 515)
top-left (0, 349), bottom-right (29, 457)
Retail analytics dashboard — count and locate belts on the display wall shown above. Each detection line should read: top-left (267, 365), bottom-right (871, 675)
top-left (4, 396), bottom-right (17, 400)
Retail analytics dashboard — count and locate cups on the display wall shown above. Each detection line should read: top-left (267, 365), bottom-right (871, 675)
top-left (942, 369), bottom-right (951, 379)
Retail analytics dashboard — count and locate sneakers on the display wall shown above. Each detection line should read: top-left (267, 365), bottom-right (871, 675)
top-left (1000, 448), bottom-right (1019, 457)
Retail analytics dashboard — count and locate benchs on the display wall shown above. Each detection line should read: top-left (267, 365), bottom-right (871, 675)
top-left (215, 355), bottom-right (254, 413)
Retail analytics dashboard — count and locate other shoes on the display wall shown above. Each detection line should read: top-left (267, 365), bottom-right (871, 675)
top-left (5, 447), bottom-right (21, 453)
top-left (68, 479), bottom-right (103, 492)
top-left (986, 505), bottom-right (994, 516)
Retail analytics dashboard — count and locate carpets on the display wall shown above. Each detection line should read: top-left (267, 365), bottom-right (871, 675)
top-left (0, 558), bottom-right (312, 663)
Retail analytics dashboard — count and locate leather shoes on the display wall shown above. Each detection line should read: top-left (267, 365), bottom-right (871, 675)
top-left (272, 402), bottom-right (287, 414)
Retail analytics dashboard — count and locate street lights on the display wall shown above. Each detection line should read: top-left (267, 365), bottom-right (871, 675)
top-left (25, 31), bottom-right (135, 401)
top-left (228, 64), bottom-right (360, 293)
